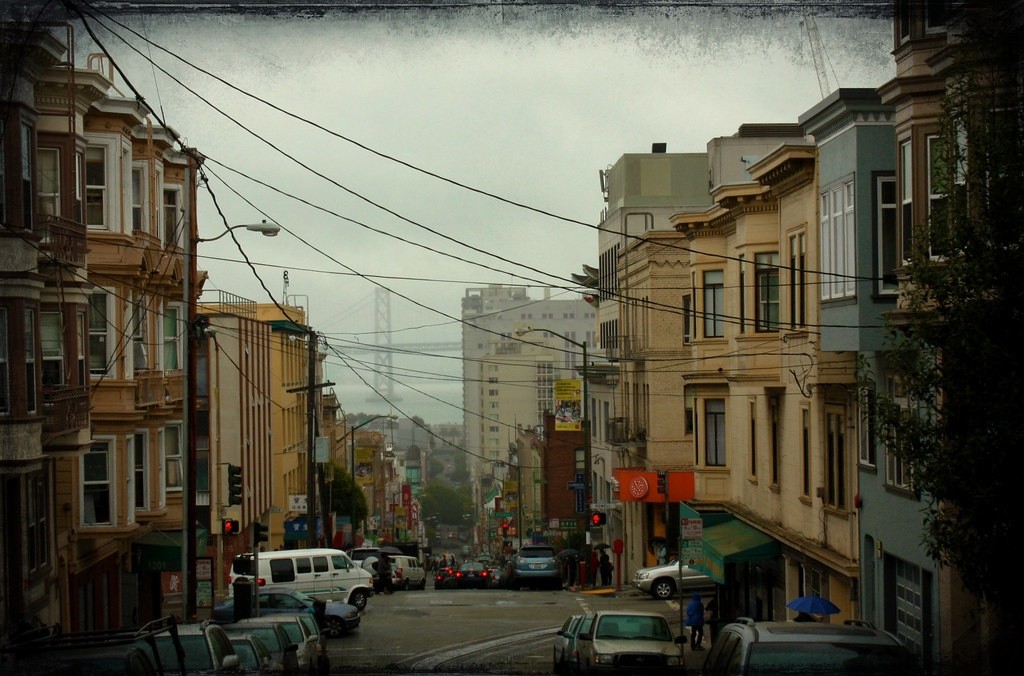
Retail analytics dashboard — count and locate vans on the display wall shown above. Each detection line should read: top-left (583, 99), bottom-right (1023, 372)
top-left (387, 555), bottom-right (426, 591)
top-left (228, 548), bottom-right (373, 611)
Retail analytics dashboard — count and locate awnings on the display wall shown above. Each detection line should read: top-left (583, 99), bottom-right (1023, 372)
top-left (689, 519), bottom-right (773, 584)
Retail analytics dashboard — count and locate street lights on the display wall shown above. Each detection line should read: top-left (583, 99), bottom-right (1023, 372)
top-left (514, 326), bottom-right (593, 591)
top-left (351, 415), bottom-right (399, 546)
top-left (487, 460), bottom-right (522, 548)
top-left (185, 220), bottom-right (282, 613)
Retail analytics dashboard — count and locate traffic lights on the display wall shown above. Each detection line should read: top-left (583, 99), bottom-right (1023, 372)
top-left (233, 557), bottom-right (251, 575)
top-left (228, 464), bottom-right (243, 506)
top-left (656, 472), bottom-right (668, 495)
top-left (223, 519), bottom-right (236, 535)
top-left (502, 522), bottom-right (509, 538)
top-left (254, 523), bottom-right (268, 548)
top-left (593, 512), bottom-right (602, 526)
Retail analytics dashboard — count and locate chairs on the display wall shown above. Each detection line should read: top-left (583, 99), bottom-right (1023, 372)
top-left (602, 622), bottom-right (619, 636)
top-left (639, 623), bottom-right (658, 636)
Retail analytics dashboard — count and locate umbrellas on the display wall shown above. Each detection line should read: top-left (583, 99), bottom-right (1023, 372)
top-left (376, 546), bottom-right (403, 553)
top-left (558, 548), bottom-right (577, 557)
top-left (594, 544), bottom-right (610, 549)
top-left (785, 596), bottom-right (840, 615)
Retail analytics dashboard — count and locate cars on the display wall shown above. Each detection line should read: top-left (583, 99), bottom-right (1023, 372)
top-left (552, 614), bottom-right (582, 676)
top-left (209, 587), bottom-right (361, 638)
top-left (577, 610), bottom-right (688, 676)
top-left (632, 555), bottom-right (717, 601)
top-left (434, 565), bottom-right (458, 589)
top-left (563, 613), bottom-right (596, 676)
top-left (474, 556), bottom-right (510, 589)
top-left (456, 562), bottom-right (494, 589)
top-left (0, 612), bottom-right (332, 676)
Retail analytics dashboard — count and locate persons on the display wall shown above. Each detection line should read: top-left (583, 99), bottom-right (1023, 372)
top-left (599, 549), bottom-right (614, 586)
top-left (451, 554), bottom-right (457, 565)
top-left (706, 594), bottom-right (717, 644)
top-left (685, 593), bottom-right (705, 651)
top-left (375, 552), bottom-right (393, 595)
top-left (590, 549), bottom-right (599, 586)
top-left (439, 556), bottom-right (449, 567)
top-left (569, 556), bottom-right (578, 592)
top-left (793, 612), bottom-right (816, 622)
top-left (431, 558), bottom-right (437, 574)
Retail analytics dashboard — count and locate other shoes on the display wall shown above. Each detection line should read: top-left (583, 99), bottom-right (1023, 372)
top-left (692, 647), bottom-right (699, 651)
top-left (696, 644), bottom-right (705, 651)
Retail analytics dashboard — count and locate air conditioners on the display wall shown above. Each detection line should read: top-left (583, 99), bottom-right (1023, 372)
top-left (609, 422), bottom-right (624, 441)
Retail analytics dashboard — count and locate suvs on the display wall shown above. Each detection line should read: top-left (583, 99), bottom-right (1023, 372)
top-left (701, 615), bottom-right (923, 676)
top-left (347, 547), bottom-right (386, 590)
top-left (509, 544), bottom-right (562, 591)
